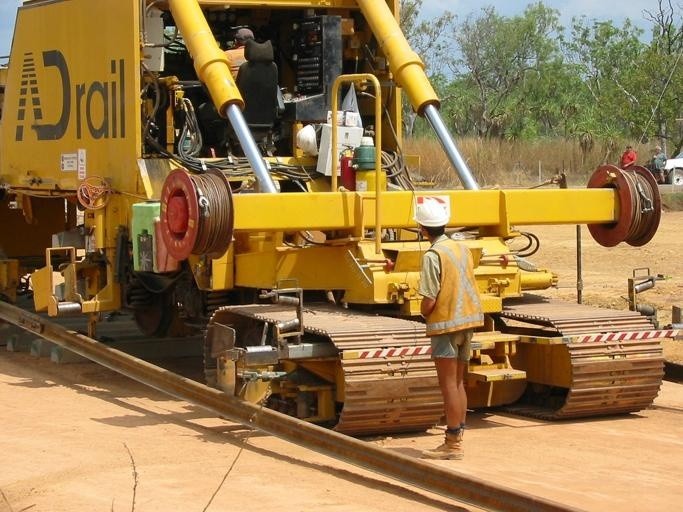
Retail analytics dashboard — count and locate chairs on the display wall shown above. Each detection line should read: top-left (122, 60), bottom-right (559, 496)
top-left (208, 41), bottom-right (278, 157)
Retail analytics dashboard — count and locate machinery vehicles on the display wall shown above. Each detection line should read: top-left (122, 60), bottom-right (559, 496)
top-left (0, 1), bottom-right (682, 435)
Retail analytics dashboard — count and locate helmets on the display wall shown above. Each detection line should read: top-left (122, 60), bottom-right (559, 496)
top-left (413, 197), bottom-right (450, 227)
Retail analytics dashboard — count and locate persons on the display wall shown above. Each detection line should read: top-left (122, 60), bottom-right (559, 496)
top-left (649, 145), bottom-right (668, 184)
top-left (621, 145), bottom-right (637, 170)
top-left (412, 195), bottom-right (484, 459)
top-left (198, 28), bottom-right (254, 158)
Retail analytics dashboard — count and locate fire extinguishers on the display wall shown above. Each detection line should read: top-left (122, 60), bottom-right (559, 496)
top-left (338, 144), bottom-right (356, 192)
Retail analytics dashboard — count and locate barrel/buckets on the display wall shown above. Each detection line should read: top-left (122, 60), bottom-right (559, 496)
top-left (353, 145), bottom-right (376, 169)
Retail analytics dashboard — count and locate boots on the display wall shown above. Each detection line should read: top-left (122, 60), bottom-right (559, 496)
top-left (422, 424), bottom-right (465, 461)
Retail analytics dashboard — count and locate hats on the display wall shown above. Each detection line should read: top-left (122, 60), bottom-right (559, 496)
top-left (234, 28), bottom-right (254, 39)
